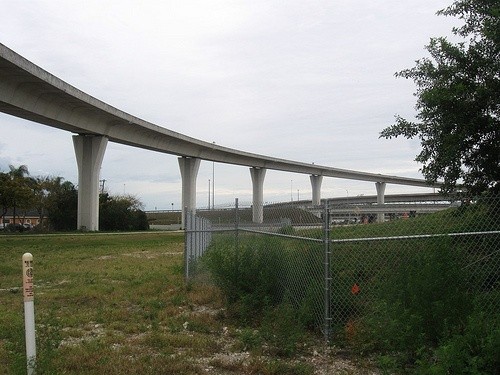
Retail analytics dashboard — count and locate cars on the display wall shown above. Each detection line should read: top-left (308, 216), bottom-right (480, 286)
top-left (1, 222), bottom-right (36, 231)
